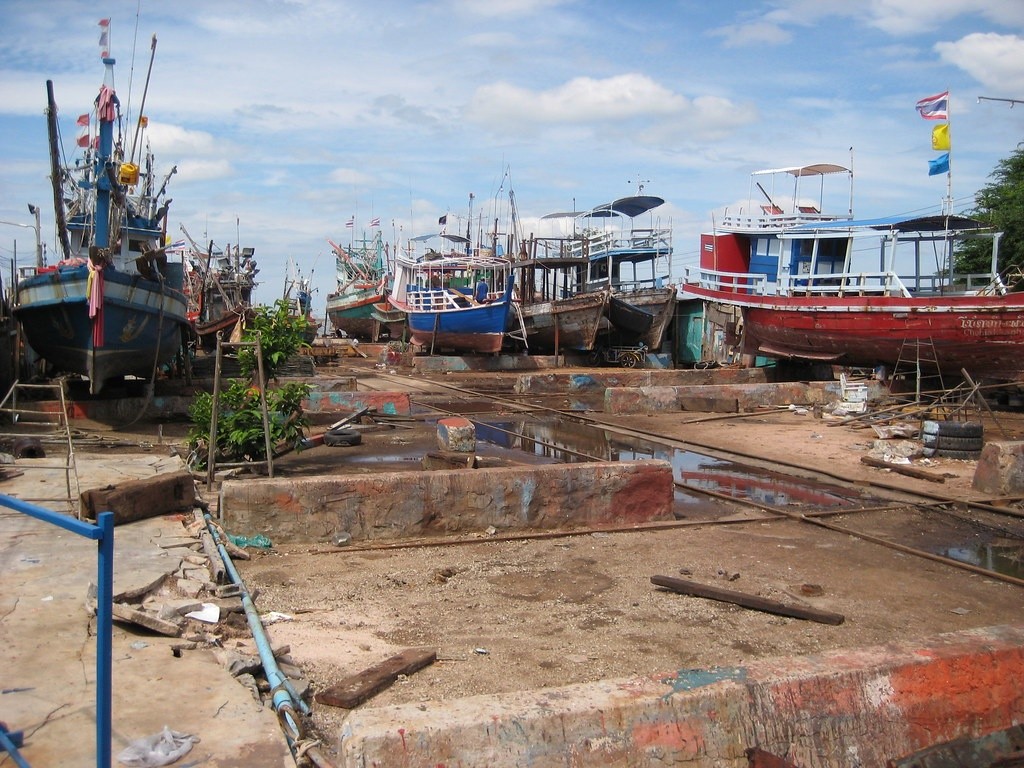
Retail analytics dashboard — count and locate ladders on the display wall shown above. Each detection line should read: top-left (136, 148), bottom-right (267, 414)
top-left (886, 336), bottom-right (948, 411)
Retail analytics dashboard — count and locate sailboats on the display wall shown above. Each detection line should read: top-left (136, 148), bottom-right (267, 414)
top-left (322, 143), bottom-right (1023, 412)
top-left (0, 0), bottom-right (318, 407)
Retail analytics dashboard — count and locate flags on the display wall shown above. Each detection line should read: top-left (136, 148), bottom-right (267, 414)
top-left (439, 216), bottom-right (447, 226)
top-left (928, 153), bottom-right (949, 175)
top-left (916, 91), bottom-right (947, 120)
top-left (76, 17), bottom-right (112, 147)
top-left (931, 125), bottom-right (950, 150)
top-left (369, 217), bottom-right (380, 227)
top-left (346, 220), bottom-right (353, 227)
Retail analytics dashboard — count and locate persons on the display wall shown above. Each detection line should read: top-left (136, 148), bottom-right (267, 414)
top-left (475, 278), bottom-right (489, 304)
